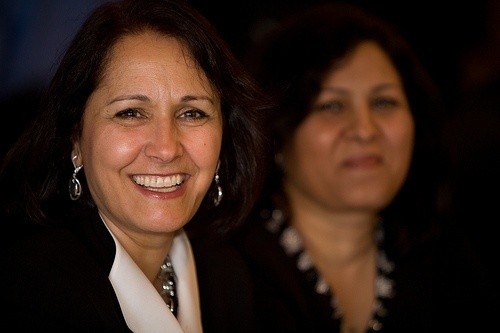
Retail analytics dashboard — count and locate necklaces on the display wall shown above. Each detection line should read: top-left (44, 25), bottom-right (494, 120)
top-left (153, 252), bottom-right (179, 319)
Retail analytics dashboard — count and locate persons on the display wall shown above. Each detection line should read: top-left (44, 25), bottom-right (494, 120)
top-left (229, 20), bottom-right (488, 333)
top-left (0, 15), bottom-right (320, 333)
